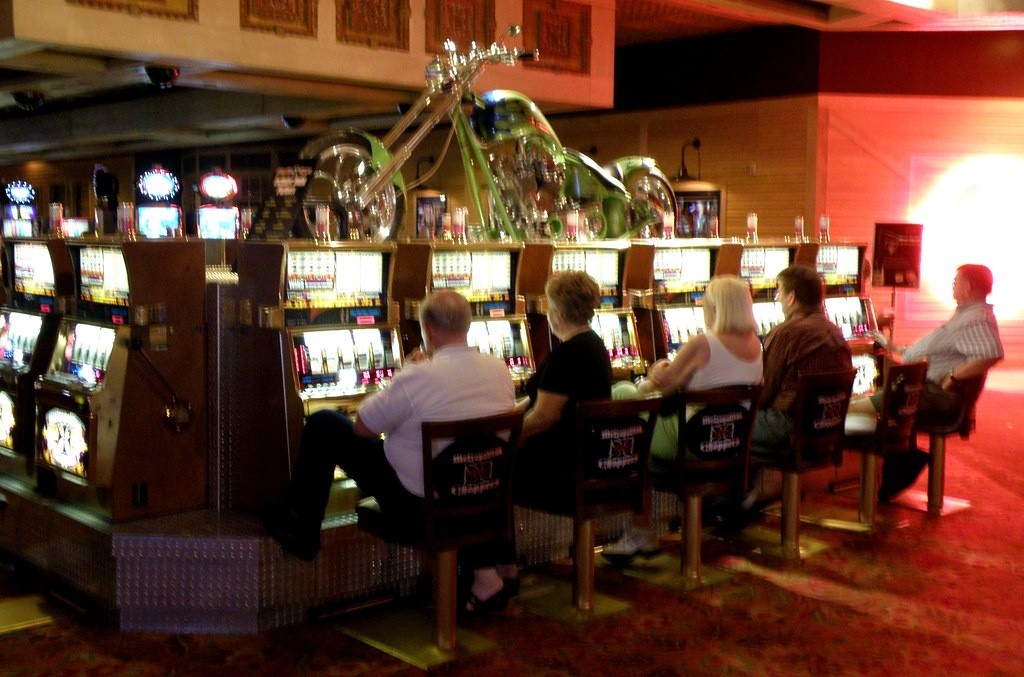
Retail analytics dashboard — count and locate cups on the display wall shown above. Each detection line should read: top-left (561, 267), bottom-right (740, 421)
top-left (442, 205), bottom-right (469, 239)
top-left (746, 212), bottom-right (757, 241)
top-left (819, 213), bottom-right (830, 241)
top-left (240, 208), bottom-right (251, 229)
top-left (566, 209), bottom-right (578, 243)
top-left (710, 215), bottom-right (719, 238)
top-left (48, 203), bottom-right (63, 234)
top-left (315, 205), bottom-right (330, 239)
top-left (117, 202), bottom-right (133, 235)
top-left (794, 215), bottom-right (804, 242)
top-left (662, 211), bottom-right (674, 239)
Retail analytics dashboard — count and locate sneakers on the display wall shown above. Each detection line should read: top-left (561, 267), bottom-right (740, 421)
top-left (600, 527), bottom-right (659, 555)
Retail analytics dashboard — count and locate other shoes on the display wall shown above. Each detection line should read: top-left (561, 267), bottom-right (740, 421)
top-left (878, 448), bottom-right (928, 502)
top-left (254, 496), bottom-right (321, 560)
top-left (501, 573), bottom-right (521, 597)
top-left (458, 583), bottom-right (507, 618)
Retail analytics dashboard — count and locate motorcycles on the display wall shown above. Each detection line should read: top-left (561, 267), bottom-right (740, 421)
top-left (294, 23), bottom-right (680, 240)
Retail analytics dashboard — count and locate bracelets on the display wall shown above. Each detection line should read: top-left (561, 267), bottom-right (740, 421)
top-left (950, 369), bottom-right (958, 383)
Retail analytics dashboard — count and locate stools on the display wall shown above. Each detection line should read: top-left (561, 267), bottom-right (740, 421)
top-left (621, 381), bottom-right (765, 598)
top-left (330, 408), bottom-right (526, 673)
top-left (797, 355), bottom-right (926, 537)
top-left (726, 365), bottom-right (860, 568)
top-left (516, 396), bottom-right (661, 630)
top-left (889, 363), bottom-right (987, 522)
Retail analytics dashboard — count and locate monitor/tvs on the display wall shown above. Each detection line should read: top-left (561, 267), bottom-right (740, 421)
top-left (286, 248), bottom-right (871, 403)
top-left (0, 243), bottom-right (129, 391)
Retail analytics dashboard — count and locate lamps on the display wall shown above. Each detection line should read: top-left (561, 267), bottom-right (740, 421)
top-left (668, 138), bottom-right (701, 183)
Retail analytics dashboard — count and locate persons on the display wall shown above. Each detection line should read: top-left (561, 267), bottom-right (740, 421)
top-left (752, 265), bottom-right (852, 457)
top-left (455, 271), bottom-right (612, 626)
top-left (846, 263), bottom-right (1006, 502)
top-left (601, 274), bottom-right (763, 557)
top-left (263, 291), bottom-right (515, 563)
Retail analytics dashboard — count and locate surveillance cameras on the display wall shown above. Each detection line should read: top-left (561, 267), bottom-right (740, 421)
top-left (398, 103), bottom-right (411, 116)
top-left (281, 115), bottom-right (305, 128)
top-left (143, 66), bottom-right (178, 87)
top-left (11, 91), bottom-right (45, 110)
